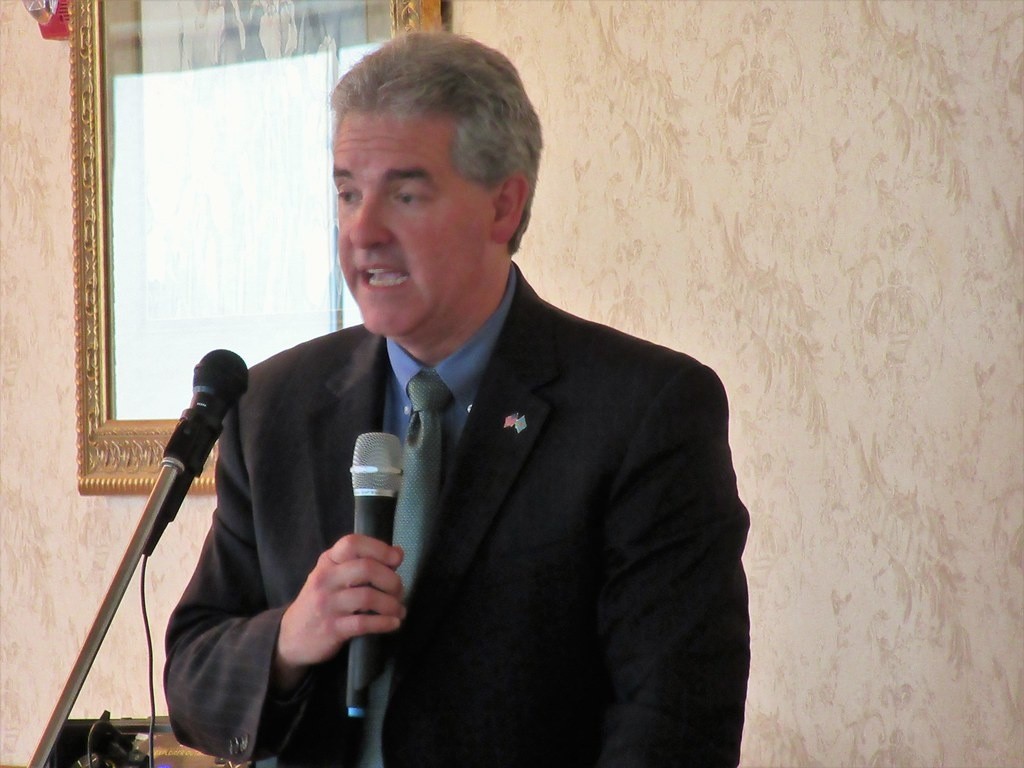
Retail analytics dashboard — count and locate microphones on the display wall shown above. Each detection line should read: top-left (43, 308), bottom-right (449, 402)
top-left (142, 349), bottom-right (249, 556)
top-left (345, 432), bottom-right (404, 718)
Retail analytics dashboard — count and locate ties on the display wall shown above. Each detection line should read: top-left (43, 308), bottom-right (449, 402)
top-left (348, 368), bottom-right (451, 768)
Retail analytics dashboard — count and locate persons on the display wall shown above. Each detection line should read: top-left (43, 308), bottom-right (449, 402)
top-left (159, 30), bottom-right (750, 768)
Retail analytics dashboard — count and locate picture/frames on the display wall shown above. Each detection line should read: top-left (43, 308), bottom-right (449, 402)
top-left (69, 1), bottom-right (446, 495)
